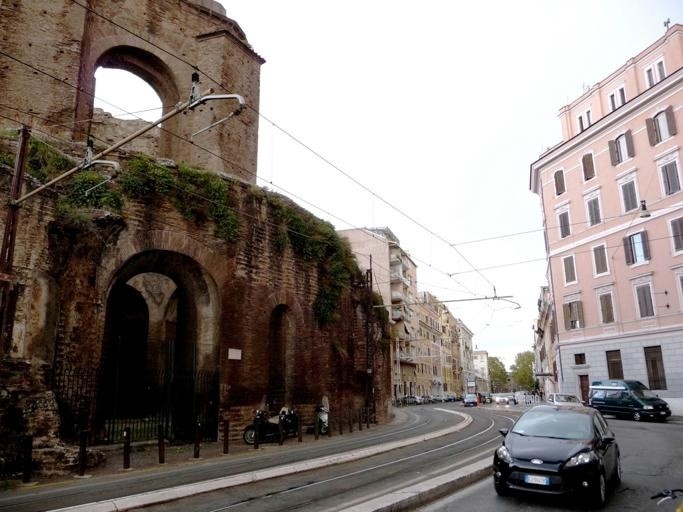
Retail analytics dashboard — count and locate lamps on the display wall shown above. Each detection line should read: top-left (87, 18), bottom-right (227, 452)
top-left (639, 199), bottom-right (650, 218)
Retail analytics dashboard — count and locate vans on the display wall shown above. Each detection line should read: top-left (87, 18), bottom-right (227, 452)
top-left (588, 378), bottom-right (673, 422)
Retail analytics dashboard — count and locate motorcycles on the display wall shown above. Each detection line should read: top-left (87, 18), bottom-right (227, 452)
top-left (313, 403), bottom-right (333, 437)
top-left (242, 408), bottom-right (311, 448)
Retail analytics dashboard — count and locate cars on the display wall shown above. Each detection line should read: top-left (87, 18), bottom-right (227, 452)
top-left (396, 395), bottom-right (461, 407)
top-left (545, 393), bottom-right (585, 408)
top-left (491, 402), bottom-right (620, 510)
top-left (474, 391), bottom-right (534, 405)
top-left (463, 393), bottom-right (479, 408)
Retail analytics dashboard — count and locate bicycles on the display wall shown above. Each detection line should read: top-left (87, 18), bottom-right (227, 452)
top-left (647, 486), bottom-right (682, 512)
